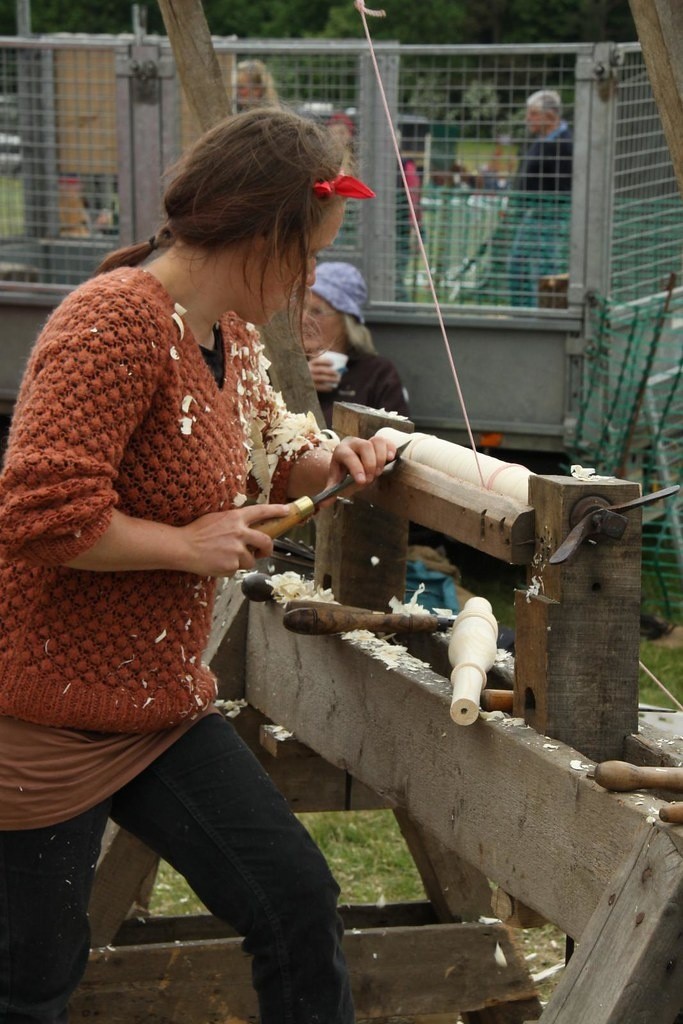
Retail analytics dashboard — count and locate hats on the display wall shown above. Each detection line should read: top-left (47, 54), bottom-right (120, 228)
top-left (309, 261), bottom-right (368, 324)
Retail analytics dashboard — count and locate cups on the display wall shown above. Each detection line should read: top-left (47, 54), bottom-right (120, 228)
top-left (319, 351), bottom-right (348, 388)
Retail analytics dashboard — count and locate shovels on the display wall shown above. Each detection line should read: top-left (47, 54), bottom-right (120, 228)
top-left (243, 436), bottom-right (411, 552)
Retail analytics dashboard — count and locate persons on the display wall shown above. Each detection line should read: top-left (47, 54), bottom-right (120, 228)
top-left (481, 134), bottom-right (516, 189)
top-left (500, 91), bottom-right (574, 308)
top-left (1, 106), bottom-right (396, 1024)
top-left (237, 63), bottom-right (280, 110)
top-left (394, 129), bottom-right (426, 303)
top-left (299, 261), bottom-right (409, 433)
top-left (326, 114), bottom-right (354, 149)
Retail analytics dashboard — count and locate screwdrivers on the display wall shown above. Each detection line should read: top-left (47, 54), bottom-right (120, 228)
top-left (480, 689), bottom-right (676, 714)
